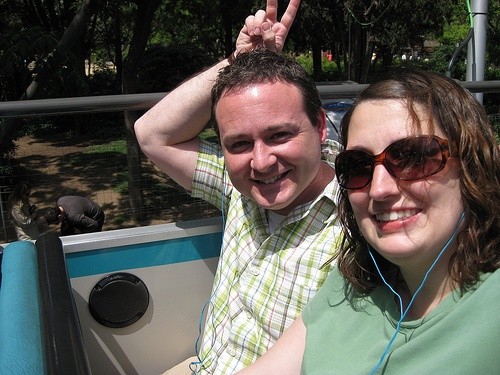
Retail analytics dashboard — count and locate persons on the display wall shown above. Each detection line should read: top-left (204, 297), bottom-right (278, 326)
top-left (229, 68), bottom-right (499, 375)
top-left (6, 182), bottom-right (43, 241)
top-left (133, 0), bottom-right (352, 375)
top-left (43, 196), bottom-right (105, 236)
top-left (368, 47), bottom-right (430, 75)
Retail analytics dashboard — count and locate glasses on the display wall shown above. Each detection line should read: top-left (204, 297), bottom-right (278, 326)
top-left (335, 135), bottom-right (459, 190)
